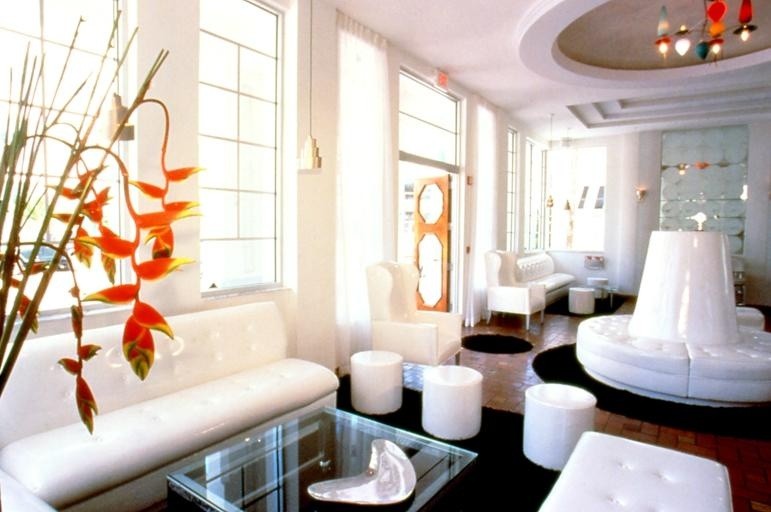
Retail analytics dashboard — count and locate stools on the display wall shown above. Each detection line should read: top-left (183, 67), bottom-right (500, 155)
top-left (351, 350), bottom-right (404, 415)
top-left (421, 365), bottom-right (482, 441)
top-left (522, 383), bottom-right (596, 473)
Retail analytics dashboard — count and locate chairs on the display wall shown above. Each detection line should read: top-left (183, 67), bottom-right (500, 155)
top-left (487, 246), bottom-right (615, 329)
top-left (364, 256), bottom-right (464, 374)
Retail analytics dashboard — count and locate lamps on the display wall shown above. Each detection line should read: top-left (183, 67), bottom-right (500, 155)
top-left (655, 0), bottom-right (758, 67)
top-left (295, 0), bottom-right (325, 175)
top-left (100, 0), bottom-right (135, 143)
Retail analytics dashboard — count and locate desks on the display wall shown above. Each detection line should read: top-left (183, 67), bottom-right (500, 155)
top-left (539, 429), bottom-right (735, 511)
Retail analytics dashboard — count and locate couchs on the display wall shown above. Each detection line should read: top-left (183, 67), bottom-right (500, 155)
top-left (567, 231), bottom-right (771, 408)
top-left (0, 301), bottom-right (344, 511)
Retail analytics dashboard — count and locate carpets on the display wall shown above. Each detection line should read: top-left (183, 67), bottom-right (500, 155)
top-left (532, 343), bottom-right (770, 436)
top-left (334, 371), bottom-right (562, 512)
top-left (462, 333), bottom-right (534, 355)
